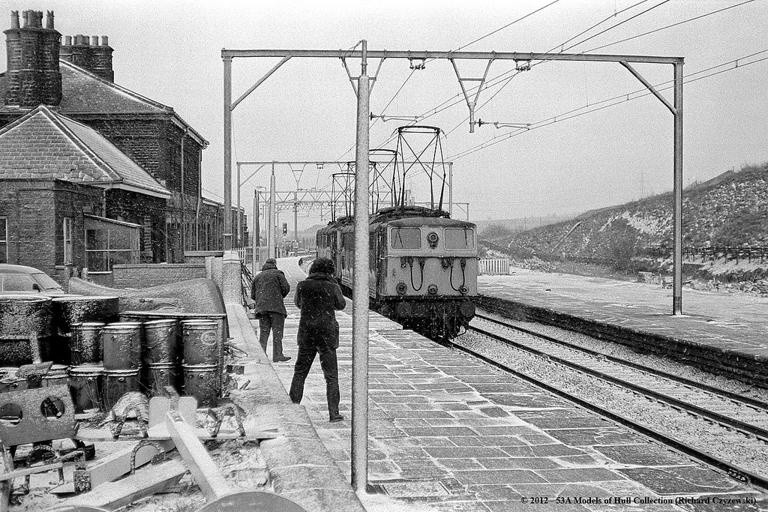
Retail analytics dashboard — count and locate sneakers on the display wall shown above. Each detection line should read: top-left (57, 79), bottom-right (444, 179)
top-left (329, 414), bottom-right (345, 423)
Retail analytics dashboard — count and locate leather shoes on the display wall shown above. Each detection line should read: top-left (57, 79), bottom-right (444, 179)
top-left (273, 354), bottom-right (292, 363)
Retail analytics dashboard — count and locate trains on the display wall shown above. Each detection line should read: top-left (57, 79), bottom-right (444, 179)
top-left (316, 206), bottom-right (478, 344)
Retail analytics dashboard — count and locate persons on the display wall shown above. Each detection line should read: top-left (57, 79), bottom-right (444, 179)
top-left (287, 256), bottom-right (344, 423)
top-left (250, 257), bottom-right (292, 362)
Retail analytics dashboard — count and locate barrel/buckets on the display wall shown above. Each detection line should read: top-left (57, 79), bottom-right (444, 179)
top-left (0, 294), bottom-right (218, 411)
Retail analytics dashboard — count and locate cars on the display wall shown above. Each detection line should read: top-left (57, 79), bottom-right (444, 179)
top-left (0, 264), bottom-right (66, 296)
